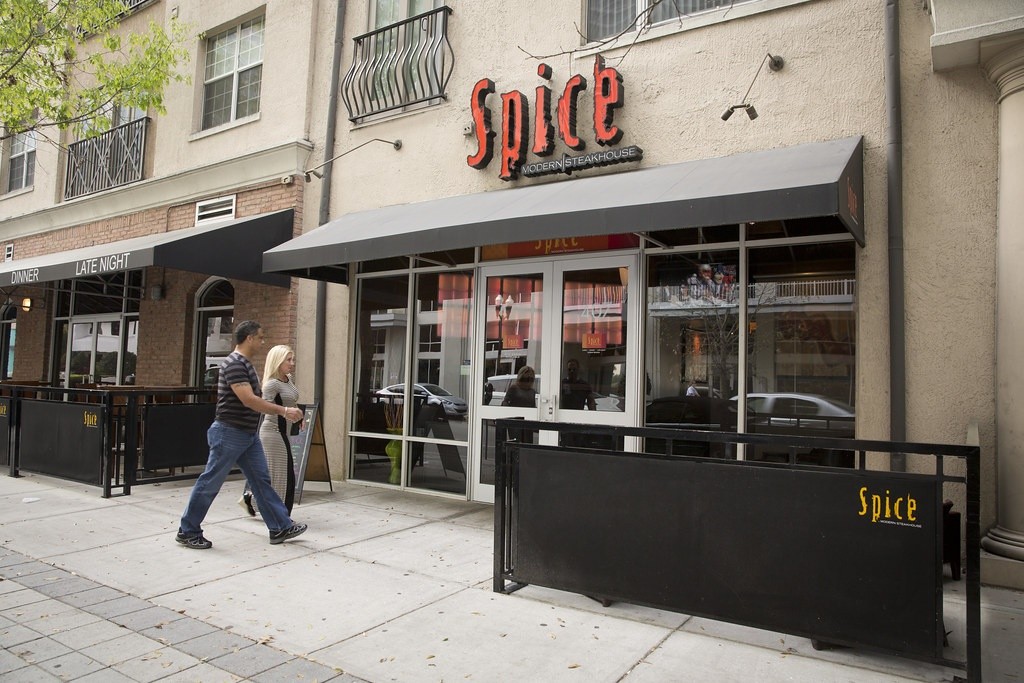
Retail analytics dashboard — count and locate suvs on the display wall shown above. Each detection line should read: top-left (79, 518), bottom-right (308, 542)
top-left (486, 373), bottom-right (624, 413)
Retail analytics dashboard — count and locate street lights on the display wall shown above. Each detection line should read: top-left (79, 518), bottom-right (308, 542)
top-left (493, 293), bottom-right (514, 375)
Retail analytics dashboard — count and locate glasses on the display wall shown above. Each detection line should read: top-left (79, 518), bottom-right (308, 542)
top-left (252, 335), bottom-right (264, 342)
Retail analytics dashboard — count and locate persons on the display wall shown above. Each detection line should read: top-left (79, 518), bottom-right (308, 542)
top-left (175, 321), bottom-right (308, 549)
top-left (497, 367), bottom-right (535, 444)
top-left (240, 345), bottom-right (305, 524)
top-left (686, 381), bottom-right (700, 396)
top-left (561, 360), bottom-right (596, 411)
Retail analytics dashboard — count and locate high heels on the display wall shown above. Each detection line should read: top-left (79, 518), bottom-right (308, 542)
top-left (239, 493), bottom-right (257, 517)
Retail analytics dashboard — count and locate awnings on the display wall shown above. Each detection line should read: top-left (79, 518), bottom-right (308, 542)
top-left (261, 136), bottom-right (864, 284)
top-left (0, 207), bottom-right (294, 289)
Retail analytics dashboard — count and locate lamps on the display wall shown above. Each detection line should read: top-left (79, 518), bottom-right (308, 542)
top-left (721, 53), bottom-right (784, 121)
top-left (151, 285), bottom-right (161, 300)
top-left (22, 298), bottom-right (31, 312)
top-left (304, 140), bottom-right (401, 182)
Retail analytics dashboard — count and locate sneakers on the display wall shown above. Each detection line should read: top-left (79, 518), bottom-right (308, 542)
top-left (174, 528), bottom-right (213, 548)
top-left (270, 524), bottom-right (308, 543)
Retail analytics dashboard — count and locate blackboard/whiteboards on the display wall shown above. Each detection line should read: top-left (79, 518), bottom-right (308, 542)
top-left (242, 402), bottom-right (333, 505)
top-left (411, 402), bottom-right (466, 482)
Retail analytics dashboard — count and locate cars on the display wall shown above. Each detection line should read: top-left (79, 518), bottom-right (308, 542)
top-left (372, 382), bottom-right (468, 420)
top-left (203, 356), bottom-right (228, 390)
top-left (643, 395), bottom-right (767, 461)
top-left (694, 386), bottom-right (721, 400)
top-left (727, 390), bottom-right (856, 468)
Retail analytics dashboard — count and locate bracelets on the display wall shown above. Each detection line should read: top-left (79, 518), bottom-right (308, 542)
top-left (283, 407), bottom-right (287, 418)
